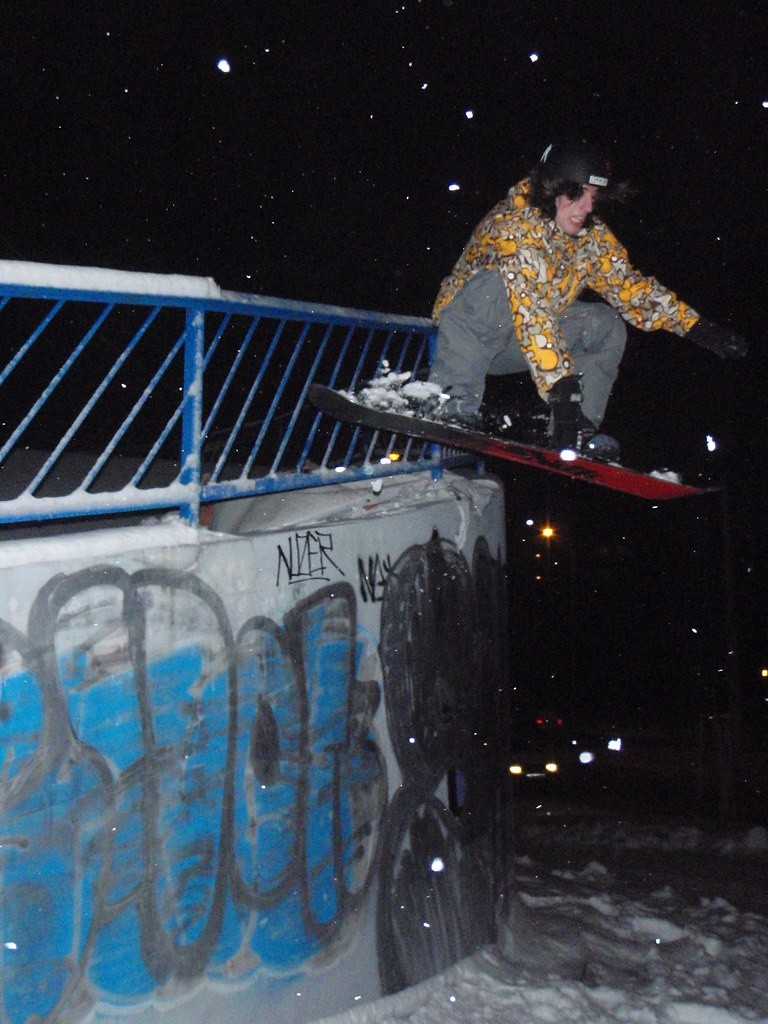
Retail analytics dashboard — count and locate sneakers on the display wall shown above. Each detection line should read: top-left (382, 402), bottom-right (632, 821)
top-left (564, 426), bottom-right (619, 461)
top-left (427, 406), bottom-right (499, 436)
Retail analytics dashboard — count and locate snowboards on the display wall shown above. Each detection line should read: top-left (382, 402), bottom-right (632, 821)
top-left (306, 379), bottom-right (723, 504)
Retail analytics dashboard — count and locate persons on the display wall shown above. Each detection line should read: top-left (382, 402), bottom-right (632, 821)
top-left (418, 136), bottom-right (750, 464)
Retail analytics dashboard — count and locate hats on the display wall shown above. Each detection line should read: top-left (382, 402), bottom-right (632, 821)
top-left (541, 134), bottom-right (609, 187)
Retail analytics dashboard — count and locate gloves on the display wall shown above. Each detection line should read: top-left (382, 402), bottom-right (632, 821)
top-left (685, 316), bottom-right (748, 361)
top-left (546, 374), bottom-right (584, 423)
